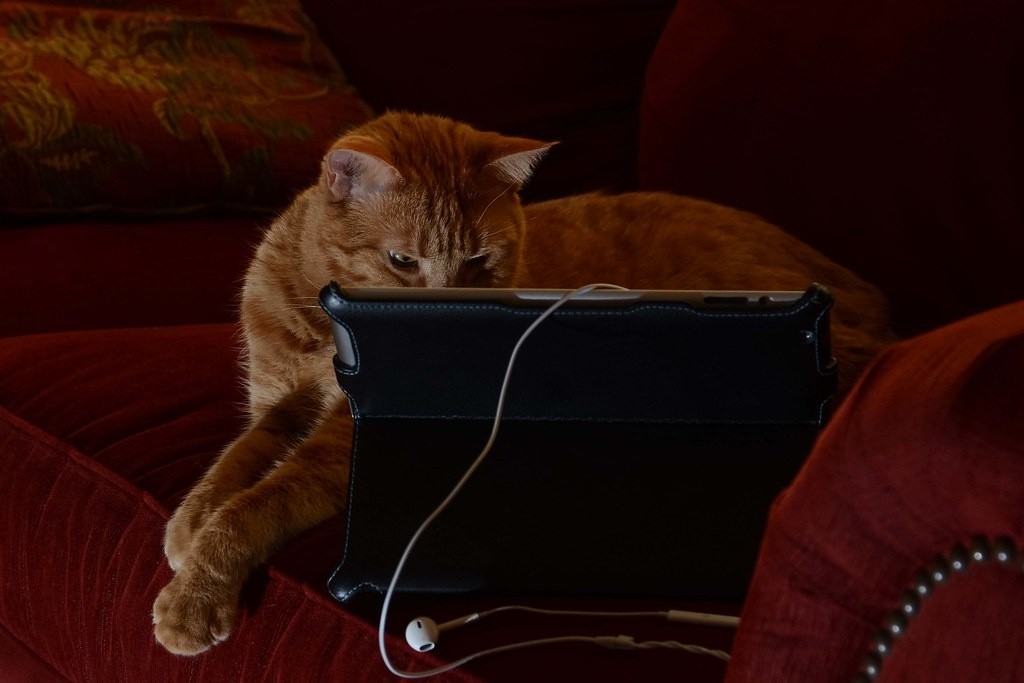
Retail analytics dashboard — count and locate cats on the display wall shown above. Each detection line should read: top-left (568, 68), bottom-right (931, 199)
top-left (149, 111), bottom-right (899, 658)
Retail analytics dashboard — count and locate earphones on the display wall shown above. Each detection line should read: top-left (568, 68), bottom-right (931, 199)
top-left (405, 612), bottom-right (479, 652)
